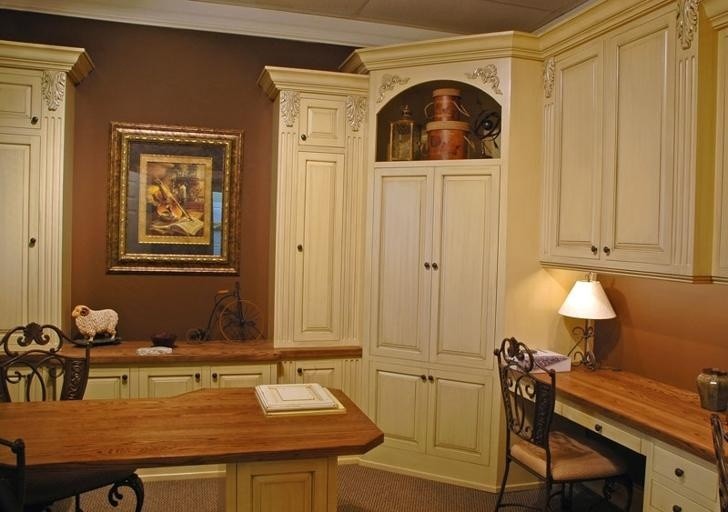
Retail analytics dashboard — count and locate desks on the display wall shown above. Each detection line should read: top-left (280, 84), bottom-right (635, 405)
top-left (0, 384), bottom-right (383, 512)
top-left (507, 363), bottom-right (727, 512)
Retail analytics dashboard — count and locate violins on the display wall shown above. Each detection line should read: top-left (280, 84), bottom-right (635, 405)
top-left (152, 178), bottom-right (183, 222)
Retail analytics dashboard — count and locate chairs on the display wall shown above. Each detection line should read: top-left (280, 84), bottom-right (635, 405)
top-left (0, 322), bottom-right (90, 402)
top-left (0, 438), bottom-right (144, 512)
top-left (495, 337), bottom-right (627, 512)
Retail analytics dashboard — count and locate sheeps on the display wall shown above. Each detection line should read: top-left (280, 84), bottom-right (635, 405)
top-left (71, 304), bottom-right (119, 342)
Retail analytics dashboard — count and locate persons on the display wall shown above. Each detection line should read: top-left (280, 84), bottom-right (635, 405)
top-left (146, 168), bottom-right (186, 220)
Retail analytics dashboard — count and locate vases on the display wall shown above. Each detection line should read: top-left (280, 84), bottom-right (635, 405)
top-left (696, 368), bottom-right (728, 412)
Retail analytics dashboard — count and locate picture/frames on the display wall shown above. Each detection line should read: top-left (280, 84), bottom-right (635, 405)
top-left (107, 121), bottom-right (245, 275)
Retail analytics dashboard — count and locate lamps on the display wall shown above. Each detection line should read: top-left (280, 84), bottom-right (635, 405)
top-left (558, 272), bottom-right (617, 372)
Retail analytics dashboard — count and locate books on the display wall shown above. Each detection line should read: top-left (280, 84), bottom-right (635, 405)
top-left (151, 215), bottom-right (204, 236)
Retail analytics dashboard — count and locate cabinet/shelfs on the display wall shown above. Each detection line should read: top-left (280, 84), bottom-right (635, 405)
top-left (256, 32), bottom-right (596, 494)
top-left (0, 40), bottom-right (95, 353)
top-left (6, 361), bottom-right (277, 482)
top-left (540, 0), bottom-right (728, 285)
